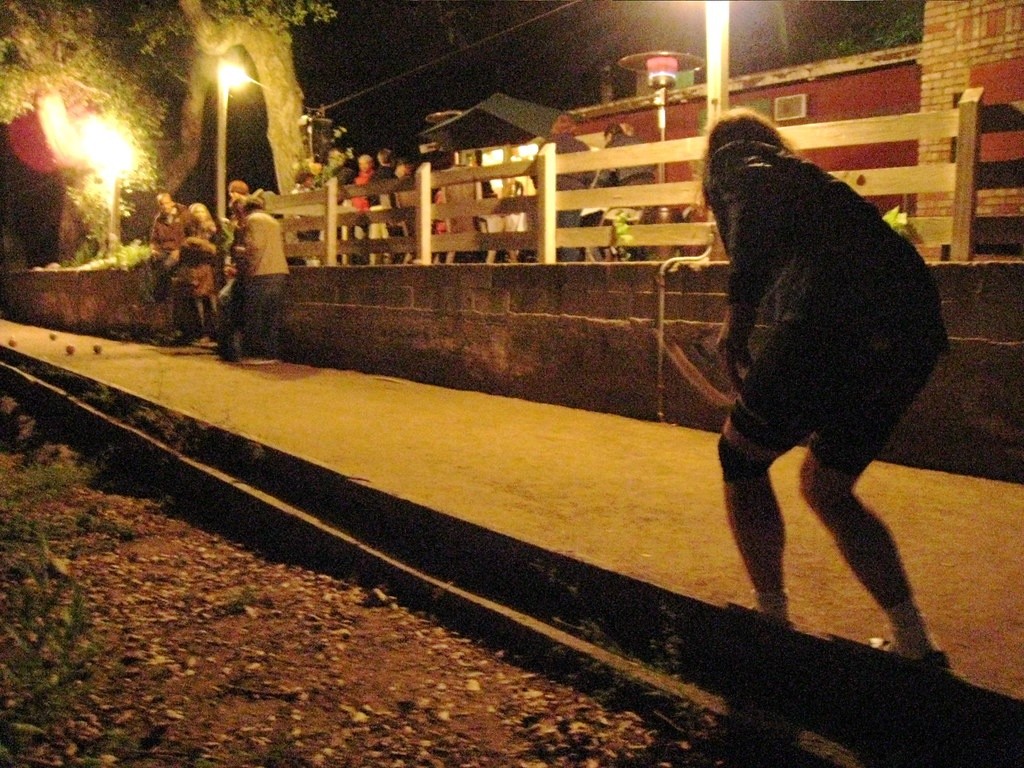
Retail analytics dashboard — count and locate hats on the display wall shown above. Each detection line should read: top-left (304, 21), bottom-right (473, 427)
top-left (293, 166), bottom-right (316, 184)
top-left (228, 181), bottom-right (249, 195)
top-left (243, 197), bottom-right (262, 210)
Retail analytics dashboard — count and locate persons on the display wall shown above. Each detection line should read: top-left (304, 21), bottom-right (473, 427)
top-left (150, 113), bottom-right (658, 364)
top-left (700, 107), bottom-right (953, 673)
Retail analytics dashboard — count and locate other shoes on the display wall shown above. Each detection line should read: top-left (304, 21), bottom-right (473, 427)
top-left (868, 637), bottom-right (953, 677)
